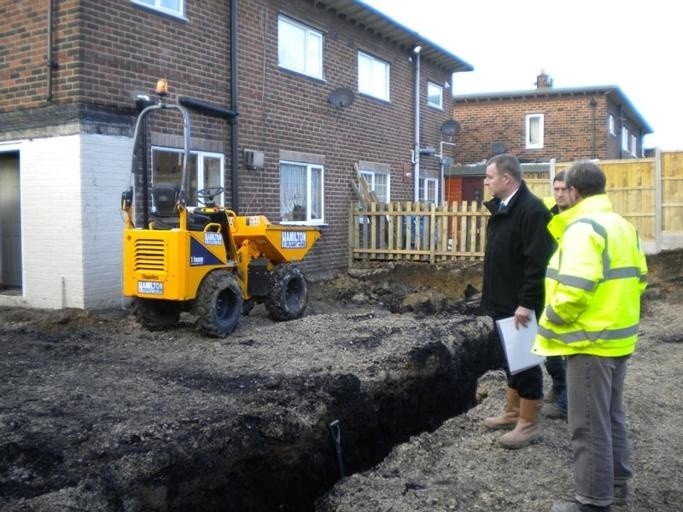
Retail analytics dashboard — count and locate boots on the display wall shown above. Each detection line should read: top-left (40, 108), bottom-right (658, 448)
top-left (483, 386), bottom-right (561, 450)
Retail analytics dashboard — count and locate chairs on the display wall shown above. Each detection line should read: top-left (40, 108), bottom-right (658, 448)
top-left (153, 185), bottom-right (210, 228)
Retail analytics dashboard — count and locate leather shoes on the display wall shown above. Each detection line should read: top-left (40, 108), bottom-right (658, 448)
top-left (550, 480), bottom-right (629, 512)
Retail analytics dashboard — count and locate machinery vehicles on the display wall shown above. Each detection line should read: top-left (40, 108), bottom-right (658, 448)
top-left (119, 76), bottom-right (323, 340)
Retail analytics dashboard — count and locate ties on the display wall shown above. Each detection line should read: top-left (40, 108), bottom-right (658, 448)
top-left (496, 202), bottom-right (506, 214)
